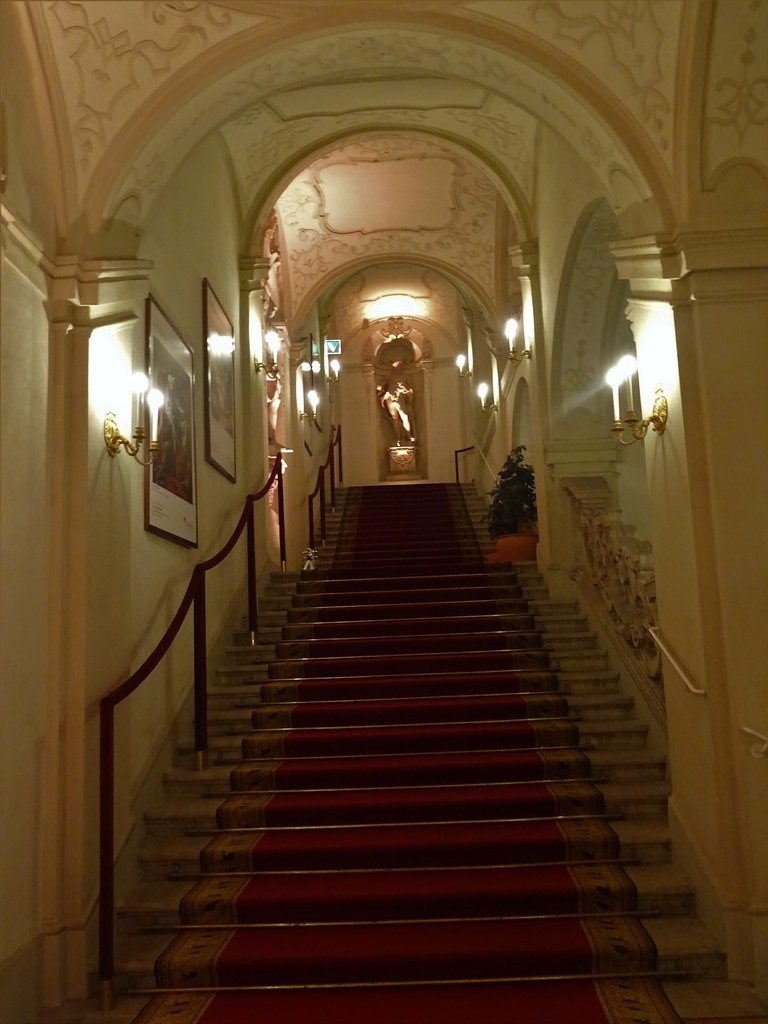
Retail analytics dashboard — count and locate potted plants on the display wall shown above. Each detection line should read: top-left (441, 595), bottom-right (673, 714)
top-left (479, 446), bottom-right (538, 564)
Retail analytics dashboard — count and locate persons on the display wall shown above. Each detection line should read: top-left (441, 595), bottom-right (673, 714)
top-left (155, 369), bottom-right (192, 490)
top-left (263, 301), bottom-right (294, 454)
top-left (377, 381), bottom-right (416, 447)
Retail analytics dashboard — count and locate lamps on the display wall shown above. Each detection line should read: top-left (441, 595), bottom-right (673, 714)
top-left (454, 354), bottom-right (471, 379)
top-left (606, 354), bottom-right (668, 446)
top-left (102, 373), bottom-right (167, 467)
top-left (254, 331), bottom-right (282, 382)
top-left (299, 391), bottom-right (319, 430)
top-left (478, 382), bottom-right (497, 411)
top-left (324, 358), bottom-right (340, 383)
top-left (504, 318), bottom-right (531, 361)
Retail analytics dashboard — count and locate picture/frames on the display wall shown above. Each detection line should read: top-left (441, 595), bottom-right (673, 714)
top-left (201, 278), bottom-right (239, 484)
top-left (144, 294), bottom-right (201, 549)
